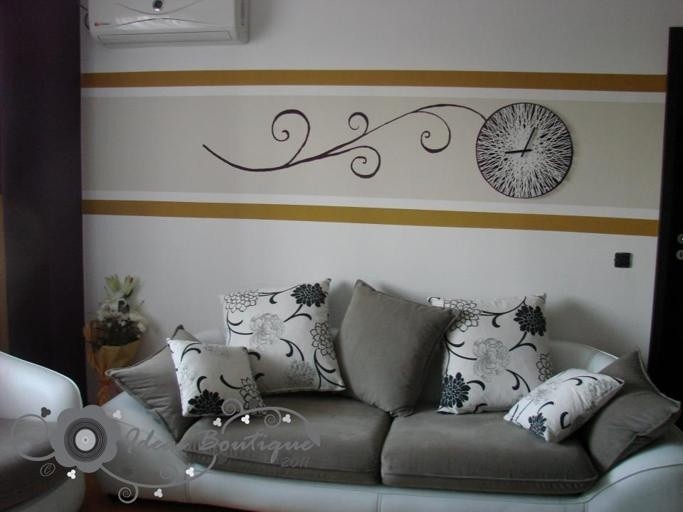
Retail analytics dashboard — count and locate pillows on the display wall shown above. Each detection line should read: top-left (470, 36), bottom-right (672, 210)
top-left (503, 368), bottom-right (624, 444)
top-left (167, 338), bottom-right (268, 418)
top-left (334, 279), bottom-right (461, 418)
top-left (572, 346), bottom-right (681, 477)
top-left (217, 277), bottom-right (347, 397)
top-left (426, 290), bottom-right (554, 415)
top-left (104, 324), bottom-right (211, 444)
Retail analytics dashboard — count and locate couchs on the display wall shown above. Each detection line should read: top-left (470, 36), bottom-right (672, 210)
top-left (94, 325), bottom-right (683, 511)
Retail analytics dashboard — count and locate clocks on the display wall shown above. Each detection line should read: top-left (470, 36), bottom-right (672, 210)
top-left (476, 102), bottom-right (573, 199)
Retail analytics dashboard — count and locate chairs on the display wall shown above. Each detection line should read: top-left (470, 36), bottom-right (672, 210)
top-left (0, 350), bottom-right (86, 512)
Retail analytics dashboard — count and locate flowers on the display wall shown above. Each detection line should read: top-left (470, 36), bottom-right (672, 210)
top-left (82, 273), bottom-right (146, 406)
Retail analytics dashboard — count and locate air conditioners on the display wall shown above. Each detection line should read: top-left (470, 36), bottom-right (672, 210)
top-left (87, 1), bottom-right (248, 43)
